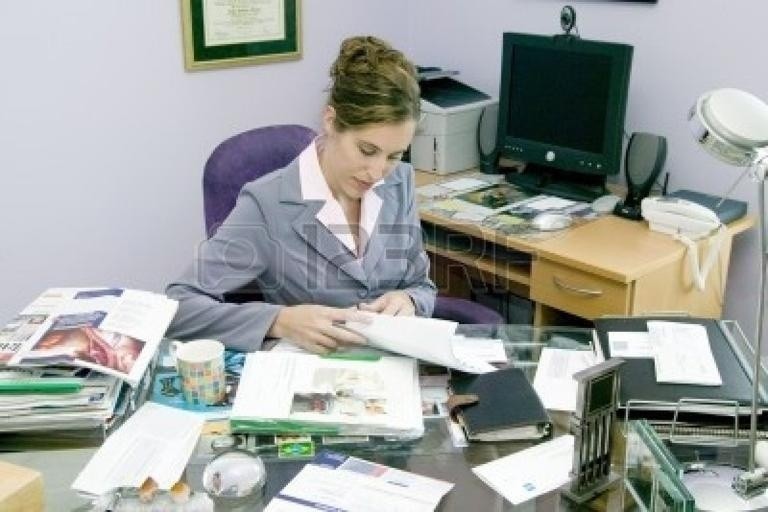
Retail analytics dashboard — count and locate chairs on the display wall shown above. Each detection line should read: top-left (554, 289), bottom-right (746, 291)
top-left (203, 123), bottom-right (503, 323)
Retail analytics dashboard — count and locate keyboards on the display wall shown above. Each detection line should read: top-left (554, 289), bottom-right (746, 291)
top-left (422, 222), bottom-right (531, 266)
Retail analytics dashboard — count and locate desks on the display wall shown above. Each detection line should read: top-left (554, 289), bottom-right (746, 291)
top-left (0, 320), bottom-right (768, 512)
top-left (413, 169), bottom-right (754, 362)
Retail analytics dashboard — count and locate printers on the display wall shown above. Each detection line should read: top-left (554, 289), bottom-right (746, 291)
top-left (398, 65), bottom-right (500, 175)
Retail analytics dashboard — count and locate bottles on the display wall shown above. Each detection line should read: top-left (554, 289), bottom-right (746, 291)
top-left (202, 450), bottom-right (268, 510)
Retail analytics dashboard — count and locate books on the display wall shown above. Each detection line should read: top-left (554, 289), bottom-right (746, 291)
top-left (0, 286), bottom-right (554, 509)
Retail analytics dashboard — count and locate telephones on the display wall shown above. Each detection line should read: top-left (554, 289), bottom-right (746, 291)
top-left (640, 196), bottom-right (720, 240)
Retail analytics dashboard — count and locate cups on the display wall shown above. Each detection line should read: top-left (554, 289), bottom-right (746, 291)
top-left (167, 339), bottom-right (226, 407)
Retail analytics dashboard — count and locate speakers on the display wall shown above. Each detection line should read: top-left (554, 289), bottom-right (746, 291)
top-left (613, 132), bottom-right (666, 220)
top-left (478, 104), bottom-right (500, 174)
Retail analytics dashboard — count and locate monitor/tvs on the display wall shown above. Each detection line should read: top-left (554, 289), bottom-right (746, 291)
top-left (497, 32), bottom-right (633, 203)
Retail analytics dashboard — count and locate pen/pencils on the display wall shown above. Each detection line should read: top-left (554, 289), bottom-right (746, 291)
top-left (662, 172), bottom-right (669, 196)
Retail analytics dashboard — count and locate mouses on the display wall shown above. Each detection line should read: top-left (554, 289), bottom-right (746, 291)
top-left (531, 212), bottom-right (572, 230)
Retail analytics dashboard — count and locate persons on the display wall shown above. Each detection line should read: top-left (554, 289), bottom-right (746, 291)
top-left (159, 33), bottom-right (440, 357)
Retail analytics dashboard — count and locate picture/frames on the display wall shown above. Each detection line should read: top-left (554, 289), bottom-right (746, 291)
top-left (181, 0), bottom-right (303, 72)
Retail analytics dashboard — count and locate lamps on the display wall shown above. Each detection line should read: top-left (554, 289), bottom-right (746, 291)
top-left (687, 88), bottom-right (768, 500)
